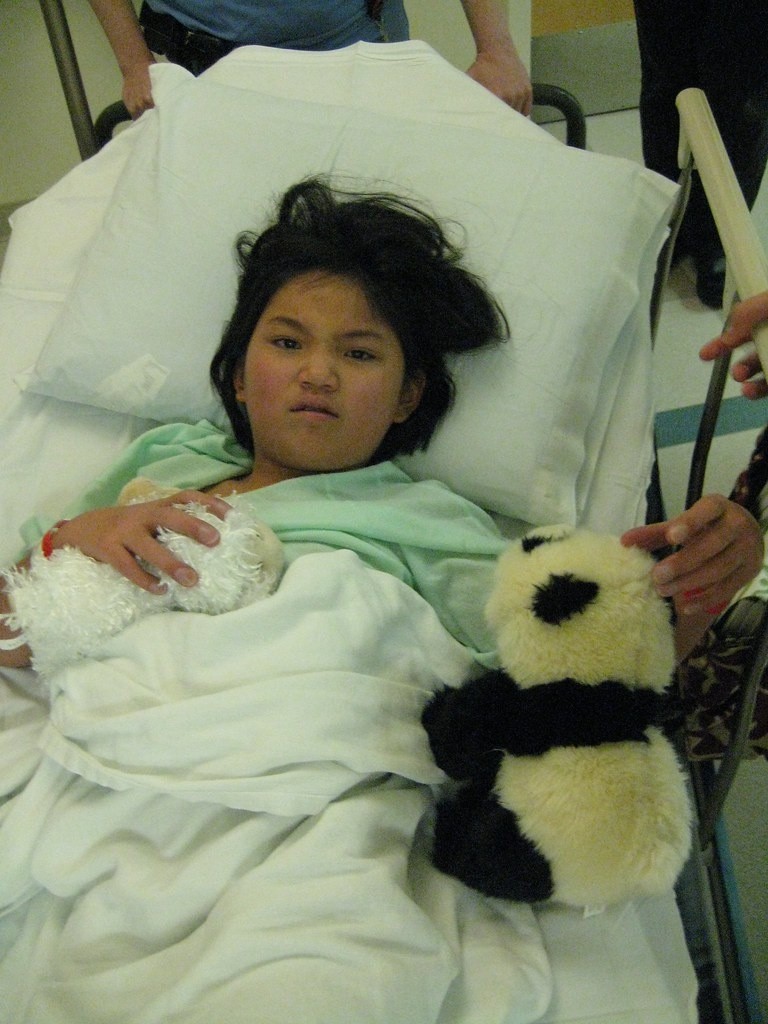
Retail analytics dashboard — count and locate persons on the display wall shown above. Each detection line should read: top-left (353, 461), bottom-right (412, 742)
top-left (89, 0), bottom-right (532, 121)
top-left (634, 0), bottom-right (768, 309)
top-left (0, 170), bottom-right (764, 674)
top-left (678, 291), bottom-right (768, 762)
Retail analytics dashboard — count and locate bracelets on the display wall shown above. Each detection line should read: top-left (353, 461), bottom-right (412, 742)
top-left (42, 519), bottom-right (70, 556)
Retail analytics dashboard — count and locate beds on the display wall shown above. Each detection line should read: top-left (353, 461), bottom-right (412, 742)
top-left (0, 41), bottom-right (768, 1024)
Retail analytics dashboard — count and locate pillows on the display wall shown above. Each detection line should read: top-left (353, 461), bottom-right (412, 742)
top-left (11, 61), bottom-right (681, 529)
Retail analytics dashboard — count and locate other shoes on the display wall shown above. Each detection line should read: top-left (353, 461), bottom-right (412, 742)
top-left (696, 229), bottom-right (725, 309)
top-left (657, 231), bottom-right (700, 268)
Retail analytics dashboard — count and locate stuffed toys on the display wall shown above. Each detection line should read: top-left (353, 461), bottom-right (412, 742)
top-left (419, 529), bottom-right (693, 906)
top-left (0, 490), bottom-right (285, 673)
